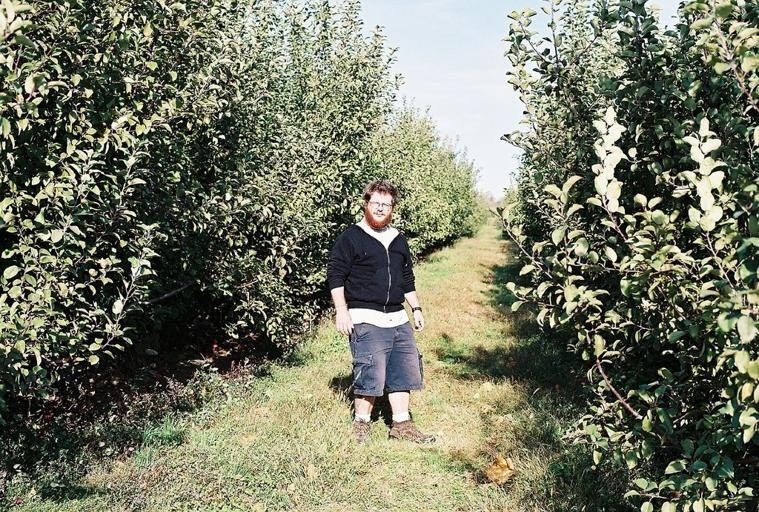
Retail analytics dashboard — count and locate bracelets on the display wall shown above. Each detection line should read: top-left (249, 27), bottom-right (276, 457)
top-left (412, 307), bottom-right (422, 312)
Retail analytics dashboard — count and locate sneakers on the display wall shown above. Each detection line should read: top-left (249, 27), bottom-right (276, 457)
top-left (389, 420), bottom-right (435, 444)
top-left (353, 419), bottom-right (370, 445)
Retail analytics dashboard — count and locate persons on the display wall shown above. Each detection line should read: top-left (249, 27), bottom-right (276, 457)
top-left (327, 181), bottom-right (435, 445)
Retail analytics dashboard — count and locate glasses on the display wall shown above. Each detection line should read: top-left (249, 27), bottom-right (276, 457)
top-left (366, 200), bottom-right (396, 210)
